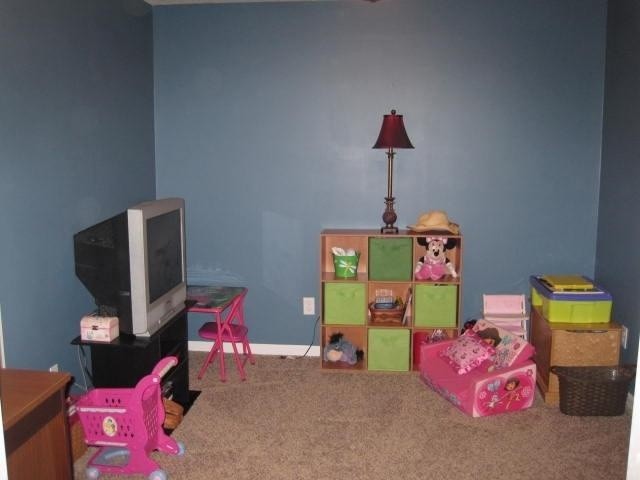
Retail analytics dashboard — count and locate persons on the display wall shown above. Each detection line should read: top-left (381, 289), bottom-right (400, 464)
top-left (502, 377), bottom-right (525, 410)
top-left (477, 327), bottom-right (502, 348)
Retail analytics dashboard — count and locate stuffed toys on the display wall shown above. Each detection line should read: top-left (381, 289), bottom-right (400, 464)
top-left (414, 237), bottom-right (457, 281)
top-left (323, 332), bottom-right (360, 365)
top-left (462, 319), bottom-right (476, 332)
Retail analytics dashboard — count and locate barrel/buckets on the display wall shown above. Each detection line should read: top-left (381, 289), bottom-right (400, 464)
top-left (330, 246), bottom-right (363, 278)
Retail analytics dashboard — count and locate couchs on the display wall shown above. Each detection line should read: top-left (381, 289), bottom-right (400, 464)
top-left (418, 318), bottom-right (539, 419)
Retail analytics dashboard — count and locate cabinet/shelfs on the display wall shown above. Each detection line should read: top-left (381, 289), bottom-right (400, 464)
top-left (70, 298), bottom-right (202, 453)
top-left (0, 367), bottom-right (76, 480)
top-left (316, 228), bottom-right (464, 376)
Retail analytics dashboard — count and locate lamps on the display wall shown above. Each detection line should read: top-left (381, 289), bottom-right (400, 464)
top-left (369, 108), bottom-right (417, 232)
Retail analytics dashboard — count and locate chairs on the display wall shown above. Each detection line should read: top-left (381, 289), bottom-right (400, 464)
top-left (197, 288), bottom-right (256, 382)
top-left (481, 292), bottom-right (531, 345)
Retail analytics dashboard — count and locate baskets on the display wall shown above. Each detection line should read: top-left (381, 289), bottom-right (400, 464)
top-left (550, 365), bottom-right (637, 416)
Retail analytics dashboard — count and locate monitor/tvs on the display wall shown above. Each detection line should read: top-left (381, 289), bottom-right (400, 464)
top-left (74, 197), bottom-right (187, 338)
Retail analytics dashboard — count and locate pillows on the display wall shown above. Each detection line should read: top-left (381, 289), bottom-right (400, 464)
top-left (436, 328), bottom-right (497, 377)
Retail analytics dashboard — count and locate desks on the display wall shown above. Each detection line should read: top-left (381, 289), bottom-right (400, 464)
top-left (186, 285), bottom-right (249, 382)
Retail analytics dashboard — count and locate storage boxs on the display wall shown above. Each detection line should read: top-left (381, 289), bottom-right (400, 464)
top-left (80, 315), bottom-right (120, 343)
top-left (527, 272), bottom-right (625, 408)
top-left (367, 300), bottom-right (405, 324)
top-left (67, 418), bottom-right (89, 465)
